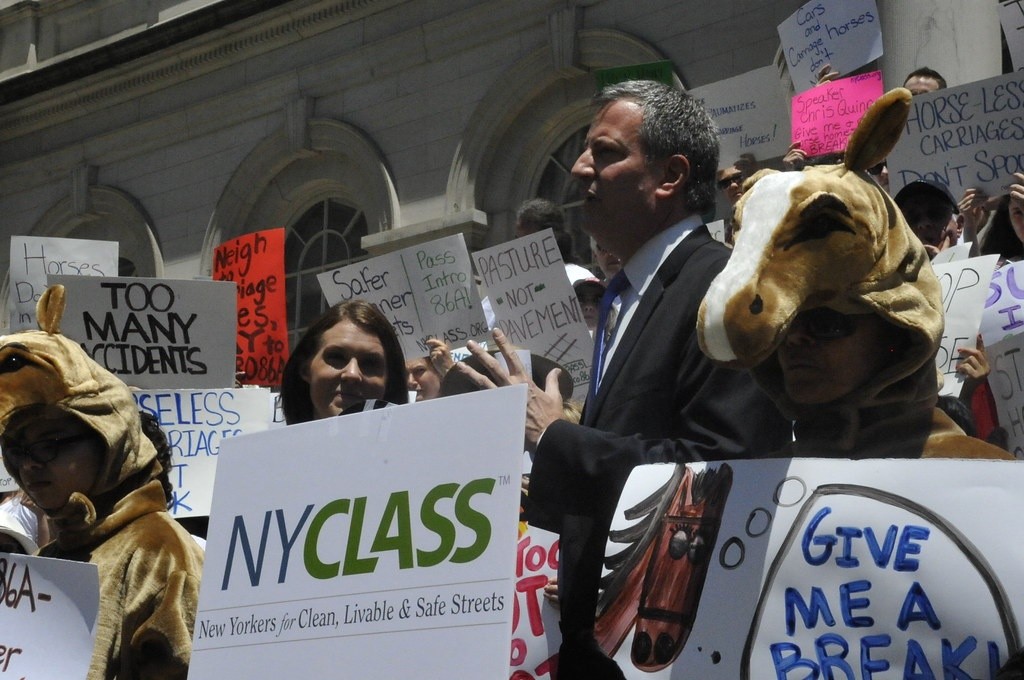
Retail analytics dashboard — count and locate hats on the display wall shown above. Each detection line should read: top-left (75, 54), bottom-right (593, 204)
top-left (894, 179), bottom-right (960, 214)
top-left (440, 349), bottom-right (574, 410)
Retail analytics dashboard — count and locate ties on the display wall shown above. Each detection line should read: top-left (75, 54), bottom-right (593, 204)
top-left (585, 269), bottom-right (630, 423)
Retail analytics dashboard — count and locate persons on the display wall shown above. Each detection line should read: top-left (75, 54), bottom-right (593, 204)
top-left (0, 200), bottom-right (621, 680)
top-left (696, 87), bottom-right (1024, 461)
top-left (457, 80), bottom-right (792, 680)
top-left (717, 66), bottom-right (1024, 453)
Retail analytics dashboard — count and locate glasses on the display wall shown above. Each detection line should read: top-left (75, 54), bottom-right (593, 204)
top-left (719, 172), bottom-right (743, 191)
top-left (867, 161), bottom-right (887, 176)
top-left (788, 304), bottom-right (856, 343)
top-left (7, 432), bottom-right (95, 468)
